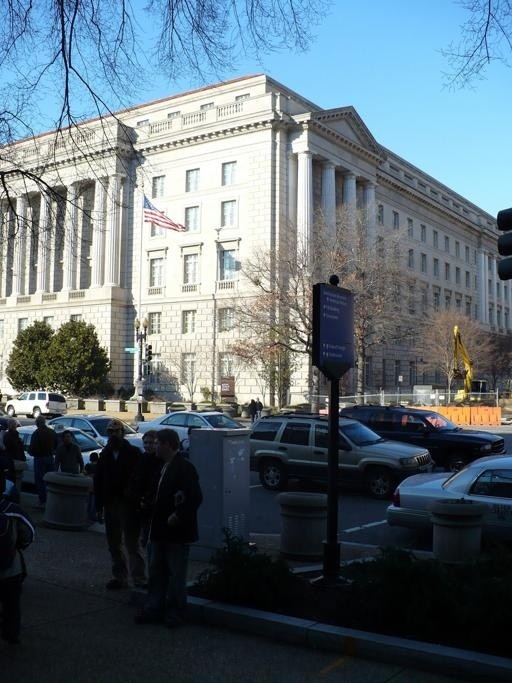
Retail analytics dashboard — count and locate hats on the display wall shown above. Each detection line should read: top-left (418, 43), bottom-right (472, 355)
top-left (106, 419), bottom-right (126, 430)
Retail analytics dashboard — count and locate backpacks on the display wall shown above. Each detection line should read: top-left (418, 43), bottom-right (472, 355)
top-left (0, 500), bottom-right (19, 573)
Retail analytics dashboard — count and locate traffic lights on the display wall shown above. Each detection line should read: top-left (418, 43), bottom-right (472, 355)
top-left (145, 342), bottom-right (153, 363)
top-left (496, 206), bottom-right (512, 283)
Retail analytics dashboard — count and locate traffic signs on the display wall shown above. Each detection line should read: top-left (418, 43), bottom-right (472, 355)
top-left (124, 347), bottom-right (138, 353)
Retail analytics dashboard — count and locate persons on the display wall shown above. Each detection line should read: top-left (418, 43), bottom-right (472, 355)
top-left (255, 397), bottom-right (263, 420)
top-left (2, 415), bottom-right (85, 514)
top-left (83, 419), bottom-right (203, 630)
top-left (2, 489), bottom-right (35, 643)
top-left (247, 399), bottom-right (257, 423)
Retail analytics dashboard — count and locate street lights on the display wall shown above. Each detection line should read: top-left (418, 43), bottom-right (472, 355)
top-left (132, 316), bottom-right (150, 419)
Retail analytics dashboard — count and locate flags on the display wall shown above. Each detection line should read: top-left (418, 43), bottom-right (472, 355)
top-left (142, 193), bottom-right (190, 233)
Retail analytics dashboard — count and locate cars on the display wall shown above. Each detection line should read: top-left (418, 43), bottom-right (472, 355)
top-left (383, 450), bottom-right (512, 547)
top-left (0, 410), bottom-right (250, 486)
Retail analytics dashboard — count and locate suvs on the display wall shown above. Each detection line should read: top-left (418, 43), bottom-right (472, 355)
top-left (246, 404), bottom-right (508, 501)
top-left (4, 389), bottom-right (68, 419)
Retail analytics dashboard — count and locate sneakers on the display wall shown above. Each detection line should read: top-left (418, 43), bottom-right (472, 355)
top-left (132, 574), bottom-right (150, 590)
top-left (105, 573), bottom-right (129, 591)
top-left (160, 610), bottom-right (182, 628)
top-left (133, 605), bottom-right (164, 626)
